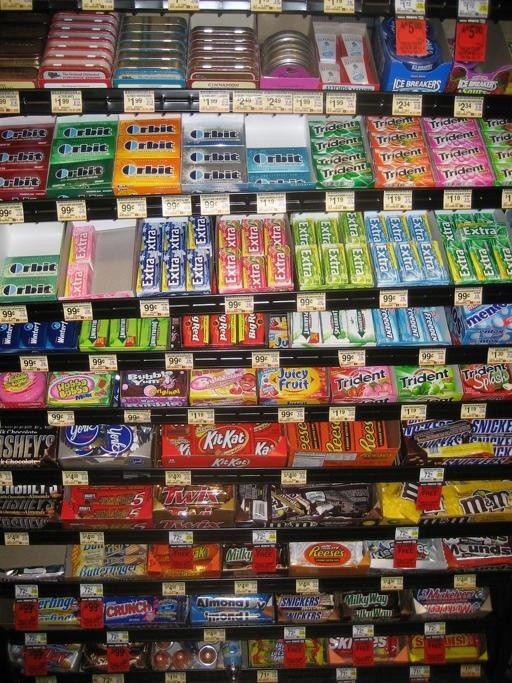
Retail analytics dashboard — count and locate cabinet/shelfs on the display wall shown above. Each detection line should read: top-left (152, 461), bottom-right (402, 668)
top-left (0, 0), bottom-right (512, 682)
